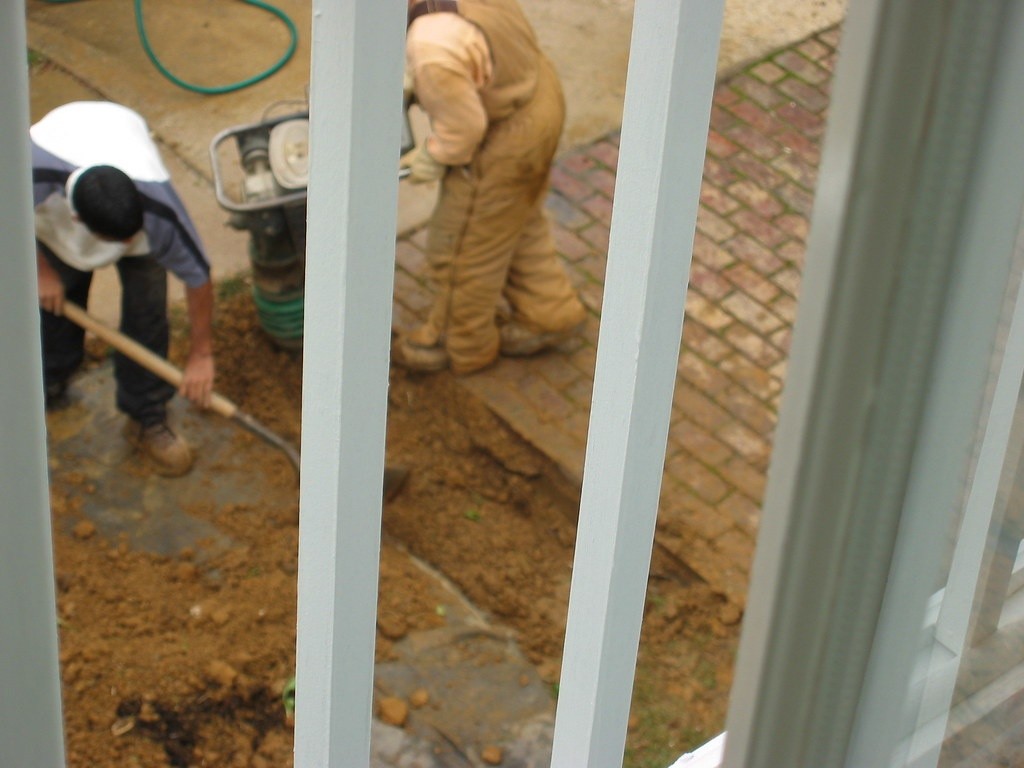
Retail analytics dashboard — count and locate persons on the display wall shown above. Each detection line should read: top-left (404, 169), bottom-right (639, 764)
top-left (399, 1), bottom-right (585, 374)
top-left (29, 101), bottom-right (215, 478)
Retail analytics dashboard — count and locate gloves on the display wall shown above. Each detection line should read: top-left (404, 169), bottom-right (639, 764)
top-left (400, 134), bottom-right (445, 186)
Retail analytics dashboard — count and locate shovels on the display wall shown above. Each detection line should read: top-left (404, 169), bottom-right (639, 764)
top-left (60, 300), bottom-right (410, 507)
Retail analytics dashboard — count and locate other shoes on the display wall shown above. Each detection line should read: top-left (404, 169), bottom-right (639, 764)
top-left (126, 411), bottom-right (191, 477)
top-left (393, 330), bottom-right (447, 370)
top-left (500, 316), bottom-right (584, 354)
